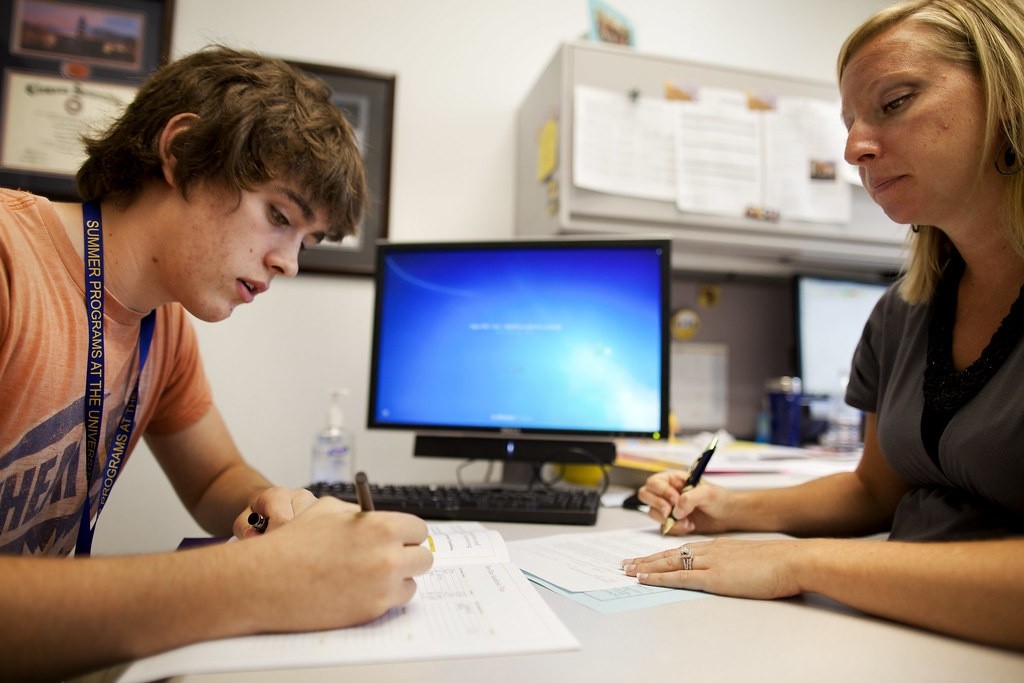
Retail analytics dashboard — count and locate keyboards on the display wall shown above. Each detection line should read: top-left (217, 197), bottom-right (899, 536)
top-left (303, 481), bottom-right (601, 527)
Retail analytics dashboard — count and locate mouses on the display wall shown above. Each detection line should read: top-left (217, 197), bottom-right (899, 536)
top-left (623, 493), bottom-right (651, 511)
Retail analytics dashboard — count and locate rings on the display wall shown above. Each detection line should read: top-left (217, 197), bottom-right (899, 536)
top-left (679, 548), bottom-right (693, 570)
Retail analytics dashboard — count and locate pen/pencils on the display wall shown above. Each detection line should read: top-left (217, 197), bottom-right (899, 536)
top-left (247, 512), bottom-right (268, 533)
top-left (355, 472), bottom-right (374, 512)
top-left (662, 435), bottom-right (719, 535)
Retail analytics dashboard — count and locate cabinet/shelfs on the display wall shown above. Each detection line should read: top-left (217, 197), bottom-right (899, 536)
top-left (517, 39), bottom-right (914, 282)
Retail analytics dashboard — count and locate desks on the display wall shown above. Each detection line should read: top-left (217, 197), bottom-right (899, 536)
top-left (161, 507), bottom-right (1024, 683)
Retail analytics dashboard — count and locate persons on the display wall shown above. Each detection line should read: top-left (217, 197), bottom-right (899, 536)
top-left (621, 0), bottom-right (1024, 653)
top-left (0, 41), bottom-right (434, 683)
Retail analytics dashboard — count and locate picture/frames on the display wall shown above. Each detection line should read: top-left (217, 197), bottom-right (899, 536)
top-left (0, 0), bottom-right (174, 204)
top-left (279, 58), bottom-right (397, 277)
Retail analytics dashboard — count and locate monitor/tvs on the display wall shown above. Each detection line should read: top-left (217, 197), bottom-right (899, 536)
top-left (364, 238), bottom-right (671, 486)
top-left (789, 273), bottom-right (895, 399)
